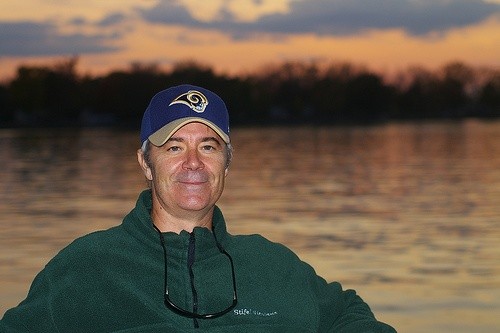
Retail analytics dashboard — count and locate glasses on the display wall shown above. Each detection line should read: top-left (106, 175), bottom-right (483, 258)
top-left (154, 224), bottom-right (239, 320)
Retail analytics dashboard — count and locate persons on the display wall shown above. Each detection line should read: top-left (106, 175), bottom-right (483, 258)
top-left (0, 85), bottom-right (398, 333)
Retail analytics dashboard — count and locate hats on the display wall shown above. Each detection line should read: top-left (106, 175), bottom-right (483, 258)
top-left (139, 84), bottom-right (231, 148)
